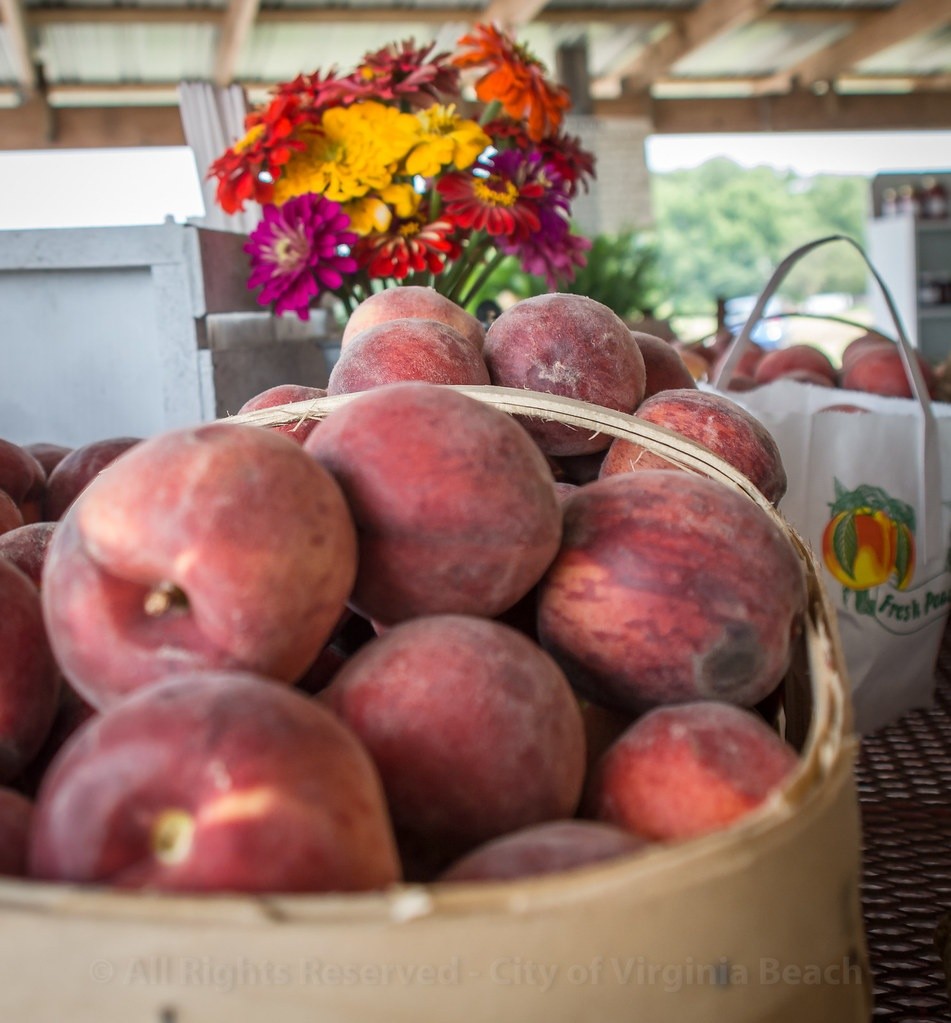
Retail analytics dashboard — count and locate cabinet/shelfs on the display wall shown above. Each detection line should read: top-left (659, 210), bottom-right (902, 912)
top-left (871, 167), bottom-right (951, 363)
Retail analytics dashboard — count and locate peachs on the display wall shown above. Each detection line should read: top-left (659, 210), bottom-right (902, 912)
top-left (2, 284), bottom-right (951, 897)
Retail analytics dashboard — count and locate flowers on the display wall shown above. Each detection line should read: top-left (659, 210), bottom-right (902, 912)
top-left (206, 21), bottom-right (601, 308)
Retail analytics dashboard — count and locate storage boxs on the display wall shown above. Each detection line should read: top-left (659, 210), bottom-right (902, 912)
top-left (0, 384), bottom-right (878, 1023)
